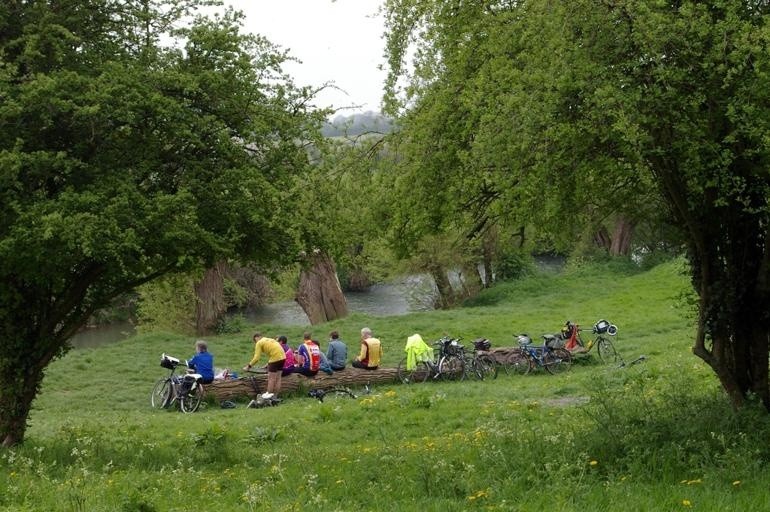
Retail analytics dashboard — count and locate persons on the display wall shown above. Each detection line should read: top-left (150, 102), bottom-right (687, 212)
top-left (275, 336), bottom-right (297, 377)
top-left (312, 340), bottom-right (329, 368)
top-left (325, 330), bottom-right (348, 371)
top-left (188, 341), bottom-right (214, 384)
top-left (293, 332), bottom-right (321, 376)
top-left (243, 333), bottom-right (286, 400)
top-left (352, 327), bottom-right (383, 370)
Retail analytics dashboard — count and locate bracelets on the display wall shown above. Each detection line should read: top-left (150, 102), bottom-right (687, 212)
top-left (249, 365), bottom-right (251, 368)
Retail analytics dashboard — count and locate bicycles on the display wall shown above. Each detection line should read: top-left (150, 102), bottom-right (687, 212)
top-left (318, 381), bottom-right (372, 402)
top-left (398, 335), bottom-right (497, 385)
top-left (152, 357), bottom-right (203, 414)
top-left (505, 319), bottom-right (647, 377)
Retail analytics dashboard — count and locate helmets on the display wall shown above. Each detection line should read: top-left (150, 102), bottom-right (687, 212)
top-left (607, 325), bottom-right (618, 336)
top-left (220, 400), bottom-right (236, 409)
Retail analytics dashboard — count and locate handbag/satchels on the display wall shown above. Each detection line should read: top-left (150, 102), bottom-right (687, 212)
top-left (475, 338), bottom-right (490, 350)
top-left (593, 319), bottom-right (610, 334)
top-left (179, 374), bottom-right (197, 396)
